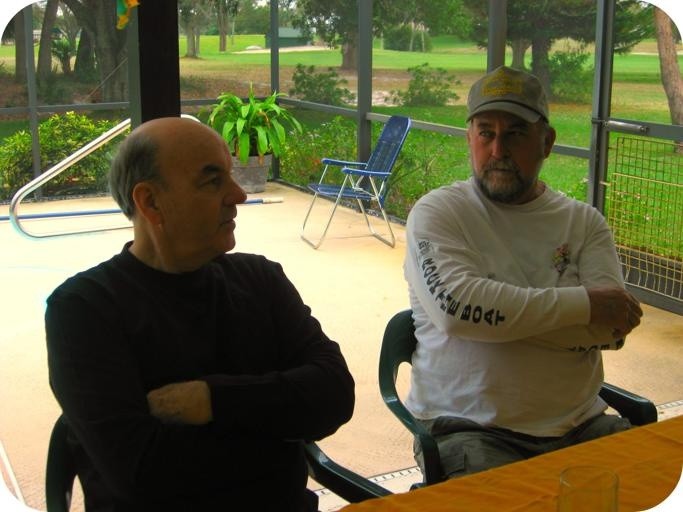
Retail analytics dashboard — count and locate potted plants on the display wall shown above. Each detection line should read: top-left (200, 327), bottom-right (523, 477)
top-left (192, 77), bottom-right (306, 196)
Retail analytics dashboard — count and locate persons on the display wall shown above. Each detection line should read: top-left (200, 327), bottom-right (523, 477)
top-left (403, 67), bottom-right (642, 487)
top-left (45, 115), bottom-right (357, 509)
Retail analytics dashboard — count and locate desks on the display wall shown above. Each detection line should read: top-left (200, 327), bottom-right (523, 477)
top-left (330, 412), bottom-right (682, 512)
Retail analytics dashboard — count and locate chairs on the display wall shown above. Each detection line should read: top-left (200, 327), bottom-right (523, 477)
top-left (299, 114), bottom-right (411, 250)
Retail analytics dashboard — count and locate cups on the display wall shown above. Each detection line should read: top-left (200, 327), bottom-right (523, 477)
top-left (555, 463), bottom-right (621, 511)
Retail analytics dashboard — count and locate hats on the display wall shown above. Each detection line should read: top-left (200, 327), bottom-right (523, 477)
top-left (466, 65), bottom-right (549, 125)
